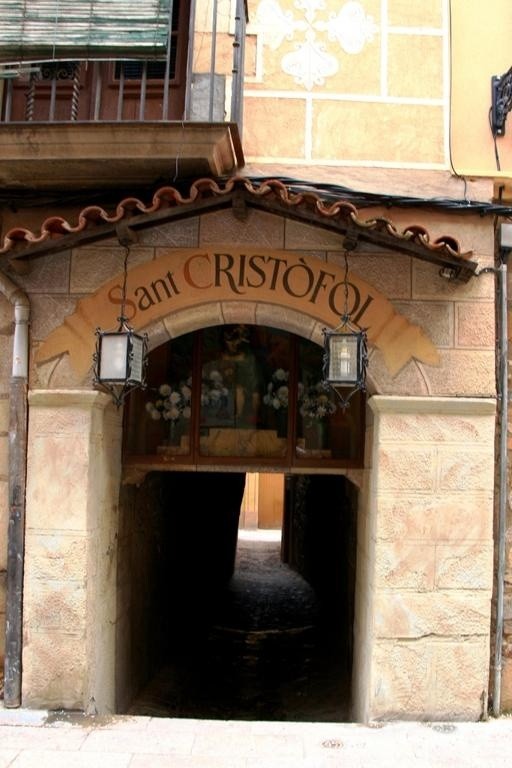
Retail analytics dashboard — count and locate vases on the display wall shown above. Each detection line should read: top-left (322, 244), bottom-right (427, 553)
top-left (276, 412), bottom-right (287, 438)
top-left (165, 420), bottom-right (184, 445)
top-left (303, 419), bottom-right (325, 449)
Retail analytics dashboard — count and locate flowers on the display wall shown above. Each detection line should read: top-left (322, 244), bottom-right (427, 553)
top-left (299, 380), bottom-right (337, 421)
top-left (146, 383), bottom-right (191, 423)
top-left (263, 368), bottom-right (289, 410)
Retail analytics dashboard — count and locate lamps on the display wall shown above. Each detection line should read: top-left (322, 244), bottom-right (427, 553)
top-left (93, 238), bottom-right (150, 409)
top-left (321, 239), bottom-right (369, 414)
